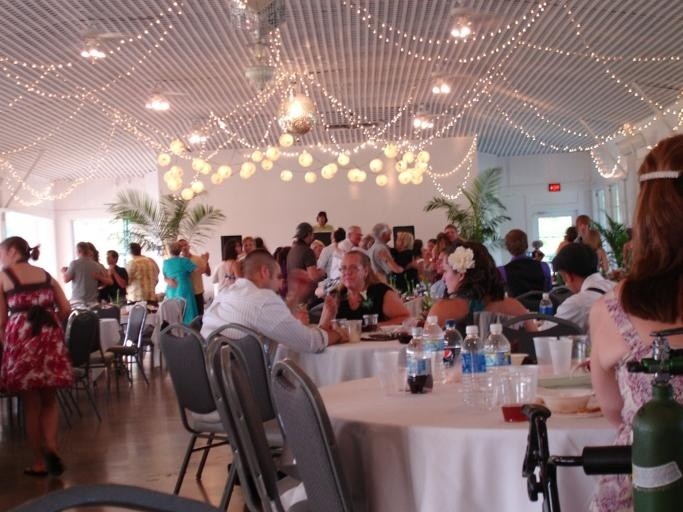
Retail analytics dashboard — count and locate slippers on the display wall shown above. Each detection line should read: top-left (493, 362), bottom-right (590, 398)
top-left (23, 465), bottom-right (49, 478)
top-left (46, 449), bottom-right (67, 477)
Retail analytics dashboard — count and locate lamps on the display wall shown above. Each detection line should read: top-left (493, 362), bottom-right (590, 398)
top-left (145, 83), bottom-right (171, 112)
top-left (80, 37), bottom-right (105, 61)
top-left (413, 108), bottom-right (434, 129)
top-left (448, 7), bottom-right (476, 39)
top-left (430, 72), bottom-right (452, 96)
top-left (277, 92), bottom-right (316, 142)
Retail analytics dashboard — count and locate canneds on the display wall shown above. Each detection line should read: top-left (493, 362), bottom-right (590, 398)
top-left (573, 333), bottom-right (587, 363)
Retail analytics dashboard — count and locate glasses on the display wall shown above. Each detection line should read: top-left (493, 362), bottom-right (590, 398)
top-left (339, 266), bottom-right (361, 273)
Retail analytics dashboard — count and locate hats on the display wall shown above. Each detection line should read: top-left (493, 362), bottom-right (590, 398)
top-left (552, 243), bottom-right (599, 277)
top-left (293, 223), bottom-right (313, 239)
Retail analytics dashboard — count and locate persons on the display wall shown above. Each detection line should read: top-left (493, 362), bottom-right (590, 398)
top-left (124, 243), bottom-right (160, 301)
top-left (539, 243), bottom-right (619, 338)
top-left (582, 229), bottom-right (609, 271)
top-left (573, 215), bottom-right (592, 243)
top-left (365, 223), bottom-right (404, 286)
top-left (61, 242), bottom-right (106, 309)
top-left (182, 248), bottom-right (350, 512)
top-left (99, 250), bottom-right (128, 305)
top-left (444, 224), bottom-right (463, 245)
top-left (242, 237), bottom-right (256, 253)
top-left (556, 227), bottom-right (578, 285)
top-left (67, 243), bottom-right (109, 298)
top-left (316, 227), bottom-right (346, 278)
top-left (309, 239), bottom-right (325, 257)
top-left (1, 239), bottom-right (74, 477)
top-left (363, 236), bottom-right (374, 249)
top-left (411, 239), bottom-right (423, 271)
top-left (285, 223), bottom-right (326, 323)
top-left (424, 241), bottom-right (539, 341)
top-left (494, 230), bottom-right (551, 314)
top-left (387, 232), bottom-right (424, 293)
top-left (310, 211), bottom-right (334, 246)
top-left (424, 233), bottom-right (444, 287)
top-left (588, 132), bottom-right (682, 512)
top-left (222, 238), bottom-right (244, 286)
top-left (255, 237), bottom-right (263, 248)
top-left (318, 250), bottom-right (410, 331)
top-left (331, 226), bottom-right (362, 278)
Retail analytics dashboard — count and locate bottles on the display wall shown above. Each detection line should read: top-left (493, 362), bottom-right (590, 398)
top-left (404, 327), bottom-right (432, 395)
top-left (440, 319), bottom-right (461, 392)
top-left (422, 314), bottom-right (445, 390)
top-left (484, 323), bottom-right (511, 411)
top-left (539, 292), bottom-right (552, 317)
top-left (461, 325), bottom-right (487, 409)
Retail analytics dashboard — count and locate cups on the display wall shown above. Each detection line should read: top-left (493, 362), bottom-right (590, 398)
top-left (344, 319), bottom-right (362, 343)
top-left (549, 339), bottom-right (572, 376)
top-left (373, 350), bottom-right (401, 398)
top-left (532, 337), bottom-right (557, 376)
top-left (561, 335), bottom-right (589, 377)
top-left (363, 314), bottom-right (378, 332)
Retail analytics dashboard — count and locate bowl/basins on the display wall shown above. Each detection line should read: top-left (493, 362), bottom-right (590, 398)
top-left (532, 387), bottom-right (597, 414)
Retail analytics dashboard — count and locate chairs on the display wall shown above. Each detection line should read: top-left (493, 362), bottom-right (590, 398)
top-left (496, 312), bottom-right (587, 362)
top-left (62, 310), bottom-right (116, 425)
top-left (203, 323), bottom-right (278, 426)
top-left (158, 322), bottom-right (225, 500)
top-left (111, 303), bottom-right (149, 389)
top-left (199, 335), bottom-right (300, 512)
top-left (266, 356), bottom-right (355, 512)
top-left (550, 285), bottom-right (573, 300)
top-left (154, 296), bottom-right (187, 372)
top-left (511, 290), bottom-right (564, 312)
